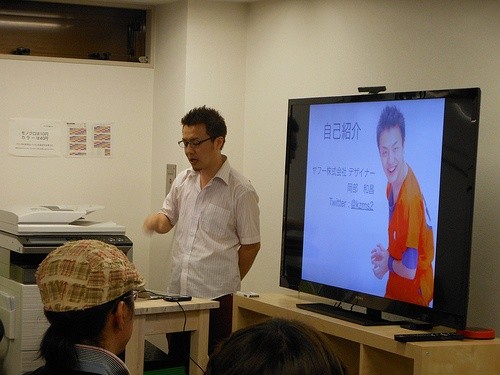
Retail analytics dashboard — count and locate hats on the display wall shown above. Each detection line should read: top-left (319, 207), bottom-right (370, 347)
top-left (34, 239), bottom-right (146, 312)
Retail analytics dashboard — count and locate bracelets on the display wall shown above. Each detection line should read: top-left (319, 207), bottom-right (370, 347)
top-left (388, 256), bottom-right (394, 271)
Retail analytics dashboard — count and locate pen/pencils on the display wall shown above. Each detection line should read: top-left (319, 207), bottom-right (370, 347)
top-left (150, 295), bottom-right (164, 298)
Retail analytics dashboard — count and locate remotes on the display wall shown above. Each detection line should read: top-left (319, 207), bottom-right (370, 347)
top-left (394, 332), bottom-right (464, 342)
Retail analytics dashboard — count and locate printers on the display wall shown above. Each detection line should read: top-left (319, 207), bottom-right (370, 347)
top-left (0, 204), bottom-right (134, 375)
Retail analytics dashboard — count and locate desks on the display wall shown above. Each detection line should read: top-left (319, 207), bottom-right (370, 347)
top-left (125, 288), bottom-right (220, 375)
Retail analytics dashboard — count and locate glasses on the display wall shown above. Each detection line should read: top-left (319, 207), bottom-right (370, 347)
top-left (111, 288), bottom-right (140, 313)
top-left (178, 134), bottom-right (216, 148)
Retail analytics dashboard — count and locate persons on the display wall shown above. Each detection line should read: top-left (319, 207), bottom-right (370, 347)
top-left (370, 107), bottom-right (434, 307)
top-left (143, 106), bottom-right (261, 358)
top-left (22, 239), bottom-right (146, 375)
top-left (206, 319), bottom-right (349, 375)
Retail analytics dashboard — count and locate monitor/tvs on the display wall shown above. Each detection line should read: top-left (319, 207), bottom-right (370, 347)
top-left (278, 87), bottom-right (482, 326)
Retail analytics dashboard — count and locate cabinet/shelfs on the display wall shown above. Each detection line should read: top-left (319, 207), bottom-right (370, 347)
top-left (232, 291), bottom-right (500, 375)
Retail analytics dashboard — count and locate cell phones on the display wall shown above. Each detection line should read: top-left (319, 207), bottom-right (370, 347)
top-left (164, 295), bottom-right (192, 302)
